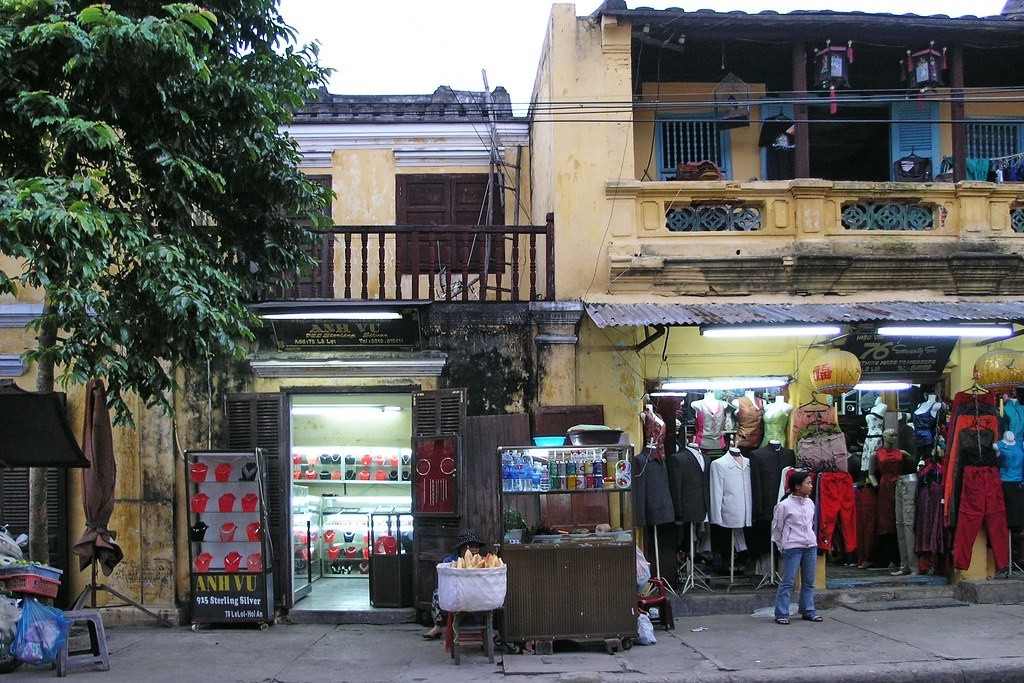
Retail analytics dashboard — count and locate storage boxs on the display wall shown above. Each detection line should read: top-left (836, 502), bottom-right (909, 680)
top-left (0, 562), bottom-right (64, 580)
top-left (0, 573), bottom-right (61, 598)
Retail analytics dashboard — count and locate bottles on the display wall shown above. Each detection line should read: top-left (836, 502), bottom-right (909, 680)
top-left (502, 450), bottom-right (551, 492)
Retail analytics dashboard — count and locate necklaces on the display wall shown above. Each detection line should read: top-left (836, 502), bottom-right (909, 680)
top-left (191, 454), bottom-right (411, 572)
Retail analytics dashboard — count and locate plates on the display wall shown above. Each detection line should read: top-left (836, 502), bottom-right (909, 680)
top-left (566, 534), bottom-right (591, 537)
top-left (591, 530), bottom-right (633, 537)
top-left (534, 534), bottom-right (562, 539)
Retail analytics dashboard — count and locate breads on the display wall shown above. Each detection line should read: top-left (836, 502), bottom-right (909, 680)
top-left (450, 549), bottom-right (504, 568)
top-left (596, 523), bottom-right (611, 532)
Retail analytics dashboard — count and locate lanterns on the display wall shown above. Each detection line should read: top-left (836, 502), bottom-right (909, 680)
top-left (812, 348), bottom-right (861, 422)
top-left (973, 348), bottom-right (1024, 395)
top-left (900, 41), bottom-right (947, 107)
top-left (815, 40), bottom-right (853, 114)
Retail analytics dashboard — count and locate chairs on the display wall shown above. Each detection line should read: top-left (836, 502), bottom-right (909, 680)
top-left (639, 579), bottom-right (678, 632)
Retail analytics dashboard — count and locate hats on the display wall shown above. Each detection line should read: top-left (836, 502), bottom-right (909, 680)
top-left (452, 529), bottom-right (486, 550)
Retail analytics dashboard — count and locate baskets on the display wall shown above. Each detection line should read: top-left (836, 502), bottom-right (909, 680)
top-left (0, 564), bottom-right (63, 599)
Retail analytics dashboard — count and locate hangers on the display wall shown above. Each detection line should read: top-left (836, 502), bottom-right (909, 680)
top-left (964, 389), bottom-right (988, 406)
top-left (791, 454), bottom-right (848, 474)
top-left (963, 377), bottom-right (988, 395)
top-left (799, 391), bottom-right (829, 414)
top-left (964, 419), bottom-right (987, 432)
top-left (901, 146), bottom-right (928, 166)
top-left (969, 452), bottom-right (994, 467)
top-left (774, 106), bottom-right (788, 119)
top-left (804, 424), bottom-right (840, 440)
top-left (808, 415), bottom-right (828, 428)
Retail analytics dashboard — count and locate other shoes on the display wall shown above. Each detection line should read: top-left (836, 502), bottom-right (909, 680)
top-left (424, 628), bottom-right (442, 639)
top-left (775, 618), bottom-right (790, 624)
top-left (802, 614), bottom-right (823, 622)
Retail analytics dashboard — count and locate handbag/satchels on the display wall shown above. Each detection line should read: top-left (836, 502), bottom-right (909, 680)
top-left (630, 608), bottom-right (656, 646)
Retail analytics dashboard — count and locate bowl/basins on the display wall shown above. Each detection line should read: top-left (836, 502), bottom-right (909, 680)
top-left (437, 562), bottom-right (507, 613)
top-left (604, 482), bottom-right (616, 488)
top-left (566, 430), bottom-right (624, 446)
top-left (533, 437), bottom-right (567, 447)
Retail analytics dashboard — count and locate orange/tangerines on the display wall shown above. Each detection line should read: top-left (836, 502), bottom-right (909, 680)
top-left (10, 560), bottom-right (49, 567)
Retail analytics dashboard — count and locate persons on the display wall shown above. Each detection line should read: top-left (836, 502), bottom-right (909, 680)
top-left (190, 522), bottom-right (209, 541)
top-left (993, 431), bottom-right (1024, 528)
top-left (845, 436), bottom-right (861, 483)
top-left (771, 472), bottom-right (823, 624)
top-left (861, 396), bottom-right (887, 471)
top-left (912, 394), bottom-right (941, 447)
top-left (898, 411), bottom-right (916, 455)
top-left (999, 398), bottom-right (1024, 441)
top-left (195, 552), bottom-right (213, 571)
top-left (421, 528), bottom-right (496, 640)
top-left (633, 389), bottom-right (795, 529)
top-left (215, 454), bottom-right (413, 574)
top-left (891, 473), bottom-right (920, 576)
top-left (191, 493), bottom-right (208, 512)
top-left (876, 428), bottom-right (911, 567)
top-left (190, 462), bottom-right (208, 482)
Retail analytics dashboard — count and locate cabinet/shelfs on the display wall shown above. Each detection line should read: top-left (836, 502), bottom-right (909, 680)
top-left (494, 437), bottom-right (633, 543)
top-left (182, 449), bottom-right (273, 576)
top-left (293, 451), bottom-right (412, 583)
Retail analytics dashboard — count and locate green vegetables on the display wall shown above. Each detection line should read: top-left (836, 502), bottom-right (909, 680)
top-left (503, 507), bottom-right (535, 543)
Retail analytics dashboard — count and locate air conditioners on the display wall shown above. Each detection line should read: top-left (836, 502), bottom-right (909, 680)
top-left (657, 374), bottom-right (793, 393)
top-left (698, 324), bottom-right (850, 335)
top-left (875, 322), bottom-right (1016, 341)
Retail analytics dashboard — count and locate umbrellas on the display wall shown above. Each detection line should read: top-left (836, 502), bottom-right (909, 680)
top-left (0, 378), bottom-right (91, 467)
top-left (82, 379), bottom-right (124, 607)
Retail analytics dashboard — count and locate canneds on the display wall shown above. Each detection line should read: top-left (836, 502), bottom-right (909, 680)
top-left (549, 460), bottom-right (603, 489)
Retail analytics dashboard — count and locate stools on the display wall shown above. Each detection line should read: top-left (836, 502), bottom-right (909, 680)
top-left (51, 606), bottom-right (111, 678)
top-left (448, 608), bottom-right (496, 667)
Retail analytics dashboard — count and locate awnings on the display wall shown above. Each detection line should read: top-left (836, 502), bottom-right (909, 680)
top-left (583, 302), bottom-right (1024, 325)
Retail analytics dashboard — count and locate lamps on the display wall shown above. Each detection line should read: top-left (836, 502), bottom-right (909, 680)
top-left (853, 378), bottom-right (914, 392)
top-left (257, 306), bottom-right (404, 320)
top-left (290, 405), bottom-right (386, 414)
top-left (386, 404), bottom-right (403, 413)
